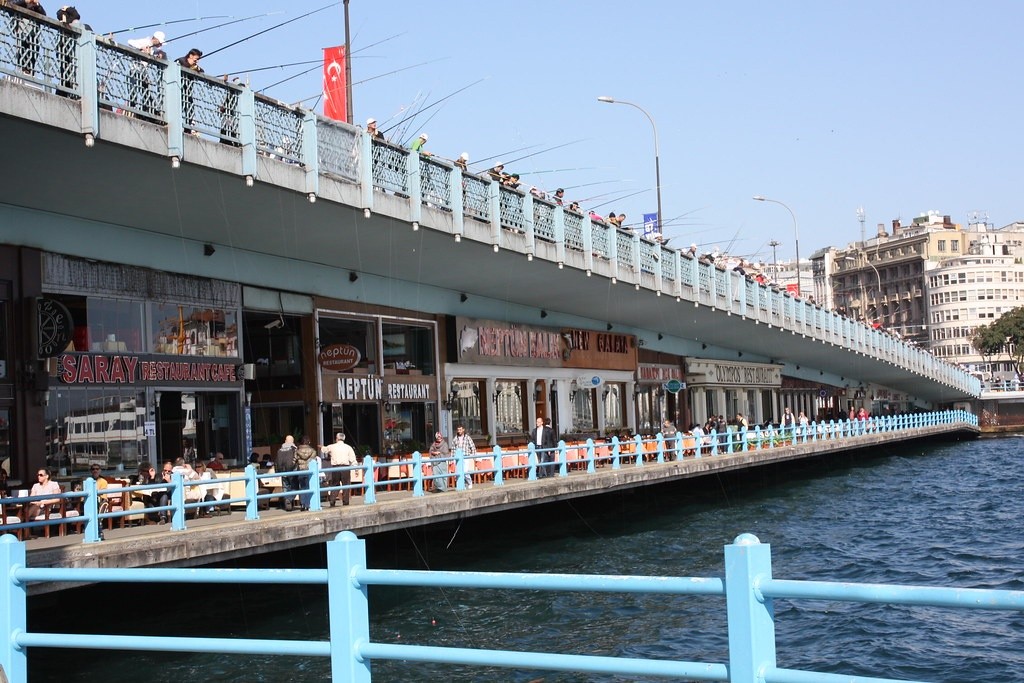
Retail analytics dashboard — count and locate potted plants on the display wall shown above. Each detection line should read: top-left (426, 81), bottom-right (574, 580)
top-left (736, 432), bottom-right (794, 451)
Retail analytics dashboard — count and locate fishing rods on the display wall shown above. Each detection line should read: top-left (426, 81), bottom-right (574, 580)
top-left (255, 30), bottom-right (409, 93)
top-left (466, 144), bottom-right (538, 166)
top-left (312, 32), bottom-right (358, 111)
top-left (621, 206), bottom-right (726, 239)
top-left (577, 189), bottom-right (628, 202)
top-left (196, 0), bottom-right (343, 61)
top-left (376, 77), bottom-right (486, 137)
top-left (290, 55), bottom-right (453, 106)
top-left (139, 11), bottom-right (284, 51)
top-left (378, 87), bottom-right (449, 147)
top-left (585, 188), bottom-right (653, 211)
top-left (98, 14), bottom-right (234, 37)
top-left (677, 238), bottom-right (745, 250)
top-left (547, 179), bottom-right (623, 193)
top-left (475, 139), bottom-right (585, 175)
top-left (506, 167), bottom-right (595, 177)
top-left (216, 54), bottom-right (386, 77)
top-left (723, 224), bottom-right (803, 286)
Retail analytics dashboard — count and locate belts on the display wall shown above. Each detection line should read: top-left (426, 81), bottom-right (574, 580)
top-left (536, 445), bottom-right (542, 448)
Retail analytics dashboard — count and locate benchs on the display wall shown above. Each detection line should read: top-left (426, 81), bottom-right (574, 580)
top-left (0, 469), bottom-right (269, 542)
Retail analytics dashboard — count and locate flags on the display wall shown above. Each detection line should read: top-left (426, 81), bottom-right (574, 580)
top-left (323, 46), bottom-right (347, 124)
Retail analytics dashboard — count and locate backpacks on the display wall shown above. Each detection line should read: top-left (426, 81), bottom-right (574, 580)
top-left (274, 449), bottom-right (297, 472)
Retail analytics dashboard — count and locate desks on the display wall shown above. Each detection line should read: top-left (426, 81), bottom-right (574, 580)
top-left (400, 450), bottom-right (529, 492)
top-left (556, 443), bottom-right (613, 472)
top-left (683, 438), bottom-right (695, 454)
top-left (619, 440), bottom-right (667, 462)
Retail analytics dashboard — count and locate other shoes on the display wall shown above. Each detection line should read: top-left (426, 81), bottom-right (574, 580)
top-left (536, 472), bottom-right (556, 479)
top-left (285, 499), bottom-right (293, 512)
top-left (468, 480), bottom-right (473, 489)
top-left (342, 501), bottom-right (349, 506)
top-left (432, 489), bottom-right (444, 493)
top-left (300, 504), bottom-right (306, 512)
top-left (158, 519), bottom-right (165, 525)
top-left (330, 501), bottom-right (335, 506)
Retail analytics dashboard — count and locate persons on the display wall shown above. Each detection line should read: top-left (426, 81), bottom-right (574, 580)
top-left (174, 49), bottom-right (205, 134)
top-left (131, 451), bottom-right (285, 525)
top-left (529, 185), bottom-right (670, 246)
top-left (54, 6), bottom-right (80, 97)
top-left (691, 414), bottom-right (719, 456)
top-left (849, 406), bottom-right (857, 421)
top-left (857, 407), bottom-right (869, 421)
top-left (440, 152), bottom-right (469, 216)
top-left (531, 418), bottom-right (558, 479)
top-left (932, 404), bottom-right (962, 412)
top-left (219, 73), bottom-right (244, 148)
top-left (451, 425), bottom-right (477, 490)
top-left (762, 417), bottom-right (774, 430)
top-left (982, 408), bottom-right (1008, 425)
top-left (881, 405), bottom-right (922, 418)
top-left (75, 464), bottom-right (108, 533)
top-left (574, 429), bottom-right (636, 442)
top-left (11, 467), bottom-right (61, 533)
top-left (488, 161), bottom-right (522, 189)
top-left (8, 0), bottom-right (46, 76)
top-left (317, 433), bottom-right (359, 507)
top-left (394, 133), bottom-right (431, 206)
top-left (678, 243), bottom-right (1024, 391)
top-left (716, 415), bottom-right (727, 455)
top-left (366, 118), bottom-right (386, 194)
top-left (868, 412), bottom-right (875, 419)
top-left (798, 412), bottom-right (809, 426)
top-left (429, 432), bottom-right (449, 493)
top-left (825, 408), bottom-right (847, 423)
top-left (291, 436), bottom-right (316, 511)
top-left (126, 31), bottom-right (166, 125)
top-left (780, 407), bottom-right (795, 427)
top-left (274, 435), bottom-right (298, 511)
top-left (736, 413), bottom-right (748, 451)
top-left (0, 467), bottom-right (12, 505)
top-left (661, 419), bottom-right (677, 461)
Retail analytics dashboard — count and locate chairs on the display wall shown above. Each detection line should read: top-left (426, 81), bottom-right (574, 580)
top-left (620, 444), bottom-right (633, 464)
top-left (565, 444), bottom-right (583, 471)
top-left (349, 446), bottom-right (424, 495)
top-left (684, 433), bottom-right (693, 457)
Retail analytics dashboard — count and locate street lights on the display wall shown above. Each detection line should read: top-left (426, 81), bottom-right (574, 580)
top-left (597, 96), bottom-right (663, 242)
top-left (753, 196), bottom-right (801, 297)
top-left (844, 256), bottom-right (882, 327)
top-left (770, 241), bottom-right (780, 283)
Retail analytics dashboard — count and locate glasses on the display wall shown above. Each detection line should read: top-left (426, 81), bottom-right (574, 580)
top-left (164, 470), bottom-right (171, 472)
top-left (37, 474), bottom-right (43, 477)
top-left (218, 458), bottom-right (223, 460)
top-left (91, 468), bottom-right (99, 471)
top-left (195, 466), bottom-right (202, 468)
